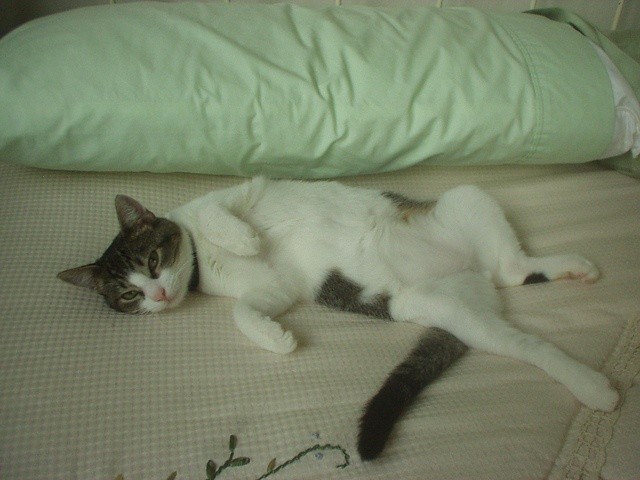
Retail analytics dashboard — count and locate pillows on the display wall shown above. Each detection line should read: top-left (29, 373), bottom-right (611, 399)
top-left (2, 2), bottom-right (640, 181)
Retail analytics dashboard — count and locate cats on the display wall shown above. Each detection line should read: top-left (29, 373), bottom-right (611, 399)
top-left (56, 174), bottom-right (621, 462)
